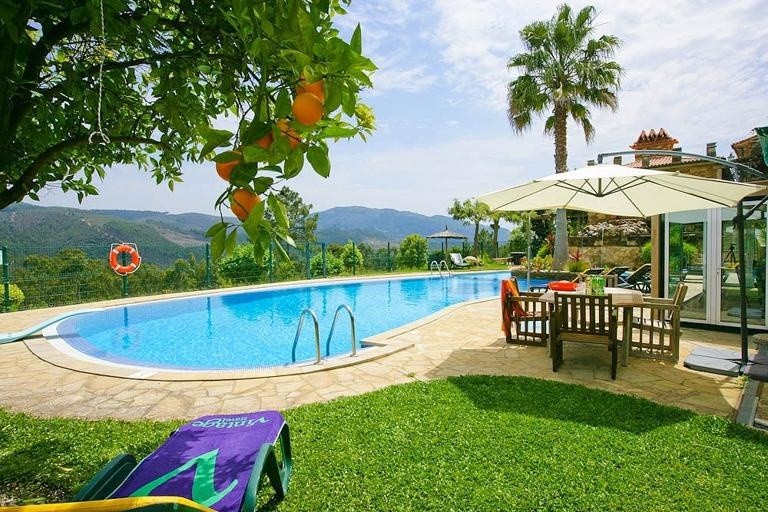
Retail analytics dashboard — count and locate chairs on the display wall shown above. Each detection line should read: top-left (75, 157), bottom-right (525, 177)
top-left (449, 252), bottom-right (471, 269)
top-left (502, 264), bottom-right (688, 380)
top-left (1, 411), bottom-right (292, 512)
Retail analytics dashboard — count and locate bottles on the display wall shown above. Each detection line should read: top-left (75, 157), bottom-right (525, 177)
top-left (574, 273), bottom-right (605, 296)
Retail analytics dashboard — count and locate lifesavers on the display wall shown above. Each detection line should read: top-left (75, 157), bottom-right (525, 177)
top-left (110, 245), bottom-right (140, 273)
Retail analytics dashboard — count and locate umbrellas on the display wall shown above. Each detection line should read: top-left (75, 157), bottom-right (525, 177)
top-left (426, 225), bottom-right (467, 263)
top-left (477, 149), bottom-right (768, 363)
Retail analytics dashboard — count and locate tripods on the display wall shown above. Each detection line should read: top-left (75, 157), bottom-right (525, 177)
top-left (697, 242), bottom-right (750, 308)
